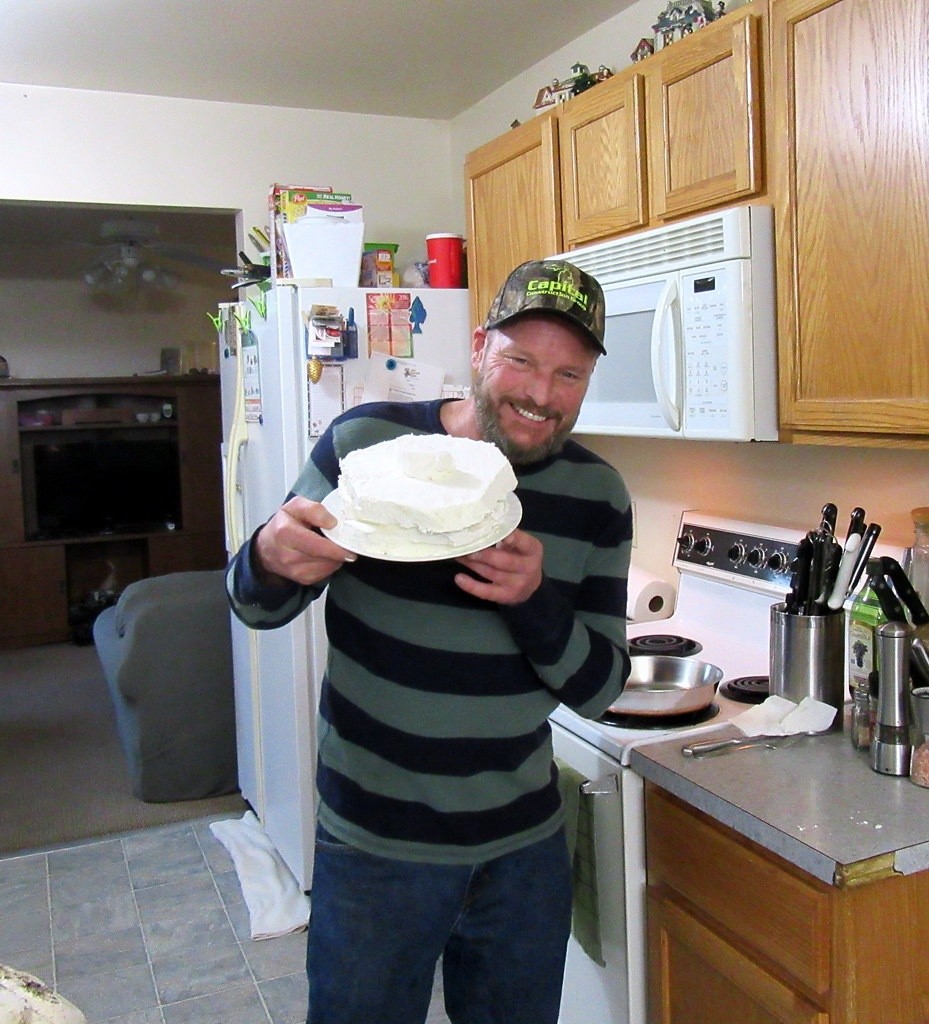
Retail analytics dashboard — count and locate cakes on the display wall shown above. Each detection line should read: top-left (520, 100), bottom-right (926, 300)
top-left (337, 433), bottom-right (518, 549)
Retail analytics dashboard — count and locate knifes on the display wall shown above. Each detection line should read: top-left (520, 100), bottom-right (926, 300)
top-left (778, 503), bottom-right (882, 614)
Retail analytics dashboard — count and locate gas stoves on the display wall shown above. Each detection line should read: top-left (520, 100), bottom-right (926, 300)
top-left (540, 505), bottom-right (904, 757)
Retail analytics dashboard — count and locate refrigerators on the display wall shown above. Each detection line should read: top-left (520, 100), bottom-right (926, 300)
top-left (215, 285), bottom-right (477, 897)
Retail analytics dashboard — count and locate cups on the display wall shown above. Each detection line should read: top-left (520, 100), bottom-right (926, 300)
top-left (911, 693), bottom-right (929, 739)
top-left (425, 232), bottom-right (466, 289)
top-left (770, 602), bottom-right (846, 736)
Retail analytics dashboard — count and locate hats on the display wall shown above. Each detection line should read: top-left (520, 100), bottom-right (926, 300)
top-left (481, 259), bottom-right (608, 355)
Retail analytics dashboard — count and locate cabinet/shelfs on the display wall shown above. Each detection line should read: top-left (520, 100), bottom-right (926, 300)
top-left (0, 372), bottom-right (228, 644)
top-left (642, 782), bottom-right (929, 1024)
top-left (461, 0), bottom-right (929, 450)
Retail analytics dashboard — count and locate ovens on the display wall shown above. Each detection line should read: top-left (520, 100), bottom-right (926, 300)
top-left (545, 720), bottom-right (648, 1023)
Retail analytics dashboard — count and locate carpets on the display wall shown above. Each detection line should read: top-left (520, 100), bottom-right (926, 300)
top-left (0, 644), bottom-right (249, 851)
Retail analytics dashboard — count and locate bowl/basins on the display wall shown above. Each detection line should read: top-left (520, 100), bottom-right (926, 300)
top-left (605, 654), bottom-right (724, 716)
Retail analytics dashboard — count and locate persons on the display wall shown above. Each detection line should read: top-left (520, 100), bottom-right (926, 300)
top-left (223, 263), bottom-right (632, 1024)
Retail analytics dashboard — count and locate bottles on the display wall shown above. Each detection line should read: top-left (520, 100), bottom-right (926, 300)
top-left (849, 557), bottom-right (907, 700)
top-left (901, 507), bottom-right (929, 658)
top-left (868, 621), bottom-right (914, 776)
top-left (850, 687), bottom-right (873, 752)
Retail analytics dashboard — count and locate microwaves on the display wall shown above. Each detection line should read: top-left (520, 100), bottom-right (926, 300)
top-left (538, 201), bottom-right (783, 445)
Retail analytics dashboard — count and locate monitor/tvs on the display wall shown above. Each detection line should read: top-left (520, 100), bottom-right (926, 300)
top-left (32, 439), bottom-right (184, 538)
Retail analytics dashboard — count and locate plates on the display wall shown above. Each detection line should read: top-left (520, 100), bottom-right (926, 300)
top-left (321, 482), bottom-right (524, 564)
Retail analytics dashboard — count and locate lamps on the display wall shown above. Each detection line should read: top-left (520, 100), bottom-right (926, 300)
top-left (80, 233), bottom-right (187, 293)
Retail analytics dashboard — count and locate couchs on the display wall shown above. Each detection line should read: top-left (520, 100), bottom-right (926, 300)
top-left (93, 568), bottom-right (238, 802)
